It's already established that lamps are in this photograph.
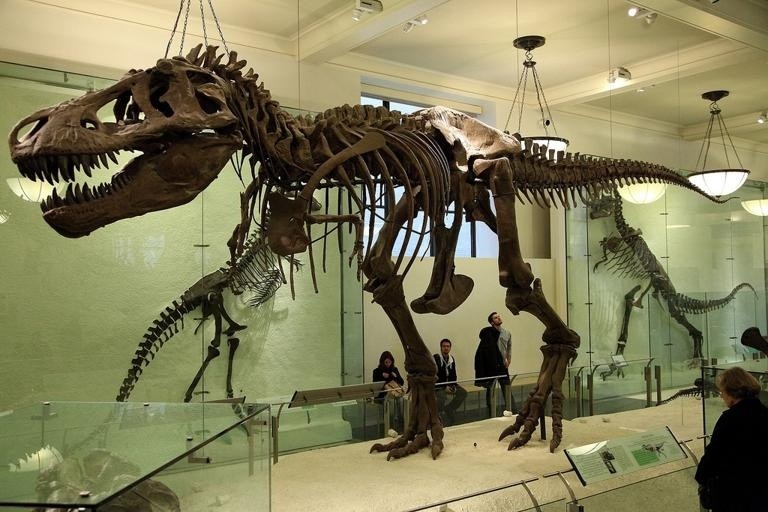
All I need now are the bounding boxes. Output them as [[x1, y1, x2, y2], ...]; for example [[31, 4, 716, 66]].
[[757, 115, 766, 124], [503, 35, 570, 163], [741, 199, 768, 216], [685, 90, 750, 196], [609, 176, 668, 204]]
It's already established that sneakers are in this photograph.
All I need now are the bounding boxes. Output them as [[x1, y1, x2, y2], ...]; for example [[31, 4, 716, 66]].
[[445, 405, 455, 421]]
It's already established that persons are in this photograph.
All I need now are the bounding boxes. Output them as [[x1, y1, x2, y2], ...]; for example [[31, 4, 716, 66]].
[[693, 366, 768, 511], [473, 312, 519, 417], [372, 350, 404, 435], [432, 339, 468, 424]]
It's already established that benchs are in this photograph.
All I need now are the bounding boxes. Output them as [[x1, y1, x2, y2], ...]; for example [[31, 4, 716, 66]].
[[364, 376, 538, 437]]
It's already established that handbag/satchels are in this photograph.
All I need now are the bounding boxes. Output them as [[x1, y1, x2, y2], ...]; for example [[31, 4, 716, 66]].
[[385, 380, 405, 397]]
